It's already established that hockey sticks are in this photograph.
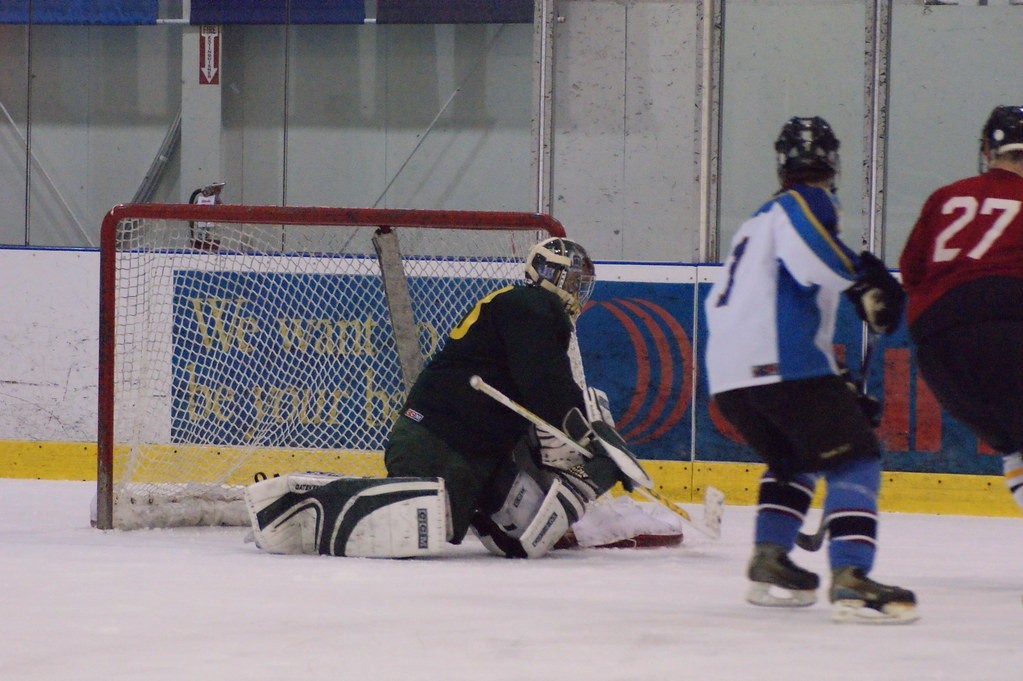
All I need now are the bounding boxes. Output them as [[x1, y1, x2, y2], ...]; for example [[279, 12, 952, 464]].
[[468, 374, 726, 542], [794, 333, 881, 553]]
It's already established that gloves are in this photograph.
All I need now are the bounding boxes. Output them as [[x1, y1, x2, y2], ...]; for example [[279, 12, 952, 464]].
[[583, 444, 637, 491], [843, 265, 902, 334]]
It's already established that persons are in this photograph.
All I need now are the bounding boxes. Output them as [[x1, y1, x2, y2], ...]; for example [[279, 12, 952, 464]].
[[899, 106, 1023, 508], [704, 115, 921, 625], [245, 237, 652, 559]]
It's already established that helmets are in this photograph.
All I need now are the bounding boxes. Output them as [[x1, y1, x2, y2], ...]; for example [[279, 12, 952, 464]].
[[525, 237, 597, 319], [775, 116, 840, 188], [978, 106, 1023, 174]]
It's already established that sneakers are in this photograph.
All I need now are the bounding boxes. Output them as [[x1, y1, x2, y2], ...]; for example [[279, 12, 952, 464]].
[[744, 543, 820, 607], [828, 568, 917, 624]]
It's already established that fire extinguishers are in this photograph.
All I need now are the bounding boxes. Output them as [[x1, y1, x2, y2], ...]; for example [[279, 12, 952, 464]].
[[188, 180, 226, 249]]
